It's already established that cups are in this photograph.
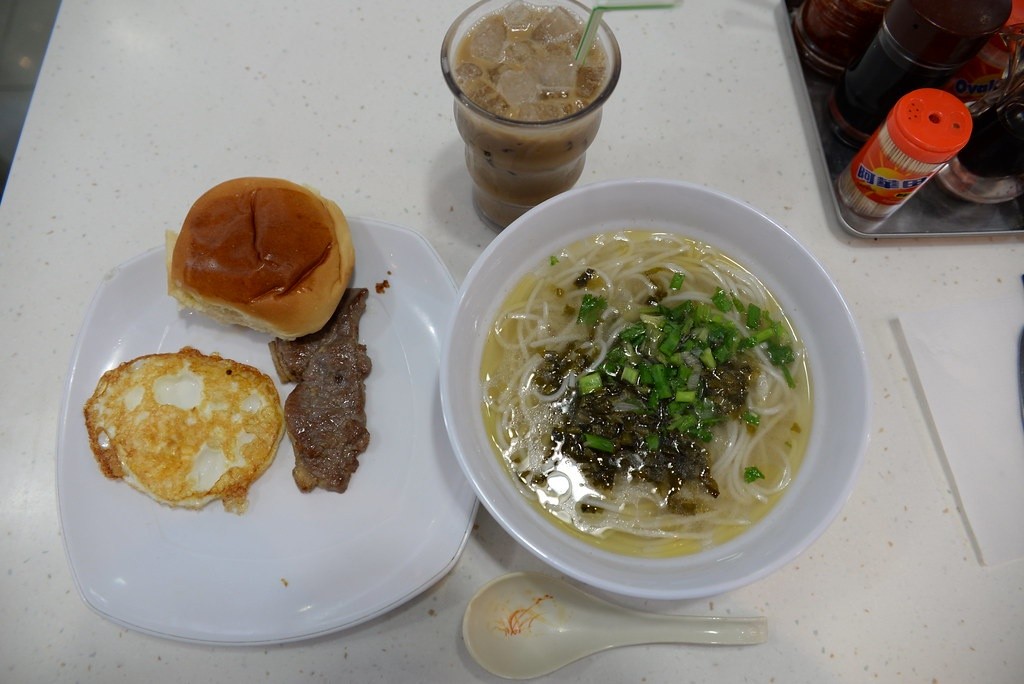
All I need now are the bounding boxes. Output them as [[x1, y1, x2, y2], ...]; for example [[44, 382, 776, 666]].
[[441, 0, 621, 229], [822, 0, 1013, 152], [793, 0, 892, 80]]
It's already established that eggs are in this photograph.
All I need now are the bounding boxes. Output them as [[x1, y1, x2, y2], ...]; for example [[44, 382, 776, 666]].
[[84, 347, 285, 510]]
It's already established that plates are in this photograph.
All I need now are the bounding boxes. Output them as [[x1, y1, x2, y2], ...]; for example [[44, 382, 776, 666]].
[[57, 217, 481, 646]]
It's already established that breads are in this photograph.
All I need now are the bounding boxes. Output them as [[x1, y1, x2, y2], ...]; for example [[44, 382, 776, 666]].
[[167, 175, 353, 337]]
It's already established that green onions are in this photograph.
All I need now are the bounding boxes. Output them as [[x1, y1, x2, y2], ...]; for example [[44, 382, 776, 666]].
[[544, 255, 797, 483]]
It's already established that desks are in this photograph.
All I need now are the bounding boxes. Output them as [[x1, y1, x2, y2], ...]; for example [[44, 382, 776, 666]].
[[0, 0, 1024, 683]]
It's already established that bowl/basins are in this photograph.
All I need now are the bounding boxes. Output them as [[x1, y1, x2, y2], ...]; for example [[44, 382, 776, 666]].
[[439, 179, 868, 600]]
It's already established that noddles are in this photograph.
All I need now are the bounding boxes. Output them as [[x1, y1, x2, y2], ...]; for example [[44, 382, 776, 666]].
[[481, 234, 812, 556]]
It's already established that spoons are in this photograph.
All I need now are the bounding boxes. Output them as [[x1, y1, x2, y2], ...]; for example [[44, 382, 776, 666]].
[[463, 572, 768, 680]]
